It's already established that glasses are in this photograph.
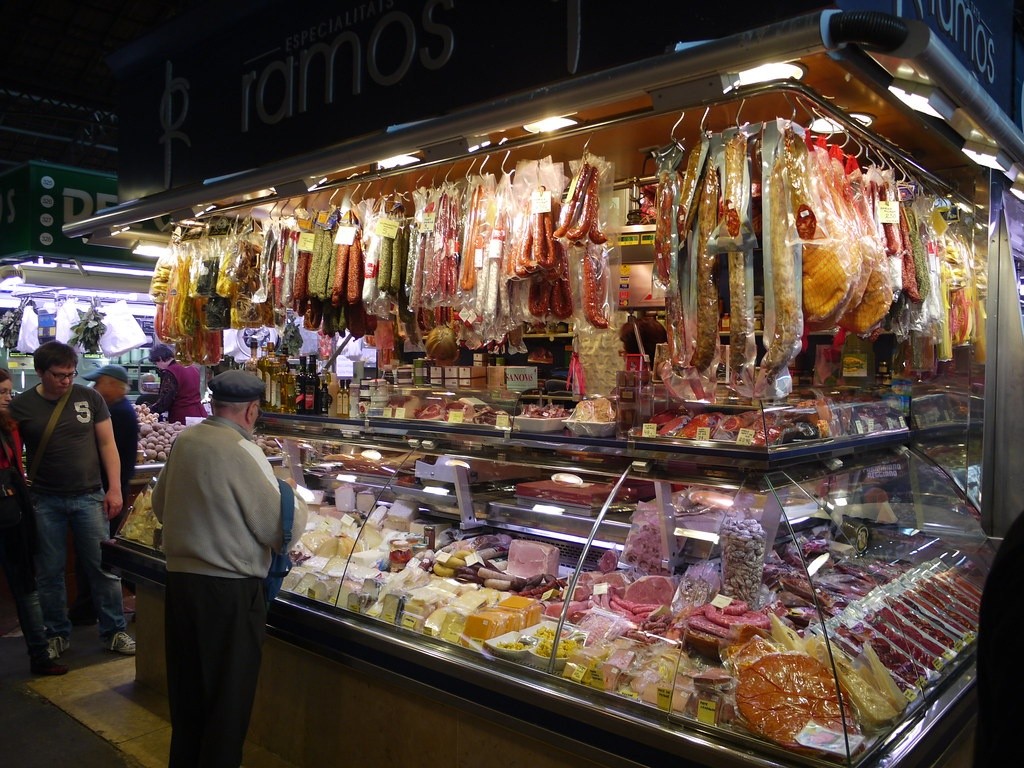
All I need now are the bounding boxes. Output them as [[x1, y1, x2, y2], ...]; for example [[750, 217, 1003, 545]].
[[618, 349, 627, 357], [47, 368, 78, 380]]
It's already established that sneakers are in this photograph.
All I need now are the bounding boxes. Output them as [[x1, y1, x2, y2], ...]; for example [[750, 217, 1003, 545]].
[[100, 631, 136, 656], [46, 636, 71, 659], [30, 658, 69, 676]]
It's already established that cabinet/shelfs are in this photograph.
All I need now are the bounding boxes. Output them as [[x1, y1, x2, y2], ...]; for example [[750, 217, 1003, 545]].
[[1, 387, 1023, 768]]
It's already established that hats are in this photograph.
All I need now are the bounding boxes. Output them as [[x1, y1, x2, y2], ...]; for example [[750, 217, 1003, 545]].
[[81, 364, 128, 385], [208, 369, 269, 405]]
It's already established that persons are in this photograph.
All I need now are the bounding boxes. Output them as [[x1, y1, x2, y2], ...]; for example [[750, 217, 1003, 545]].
[[135, 372, 169, 422], [0, 367, 70, 676], [146, 344, 210, 426], [80, 364, 142, 612], [146, 370, 291, 768], [8, 341, 139, 656]]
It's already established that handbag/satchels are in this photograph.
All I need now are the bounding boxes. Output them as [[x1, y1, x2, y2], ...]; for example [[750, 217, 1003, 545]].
[[0, 432, 22, 533]]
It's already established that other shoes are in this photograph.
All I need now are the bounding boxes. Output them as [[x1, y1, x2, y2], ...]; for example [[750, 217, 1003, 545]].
[[131, 613, 136, 623], [72, 613, 98, 626]]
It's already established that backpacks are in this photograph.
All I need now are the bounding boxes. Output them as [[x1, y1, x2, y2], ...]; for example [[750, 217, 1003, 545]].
[[263, 476, 296, 613]]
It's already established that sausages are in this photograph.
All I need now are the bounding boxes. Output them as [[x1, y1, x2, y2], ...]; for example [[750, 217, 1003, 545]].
[[153, 117, 988, 761]]
